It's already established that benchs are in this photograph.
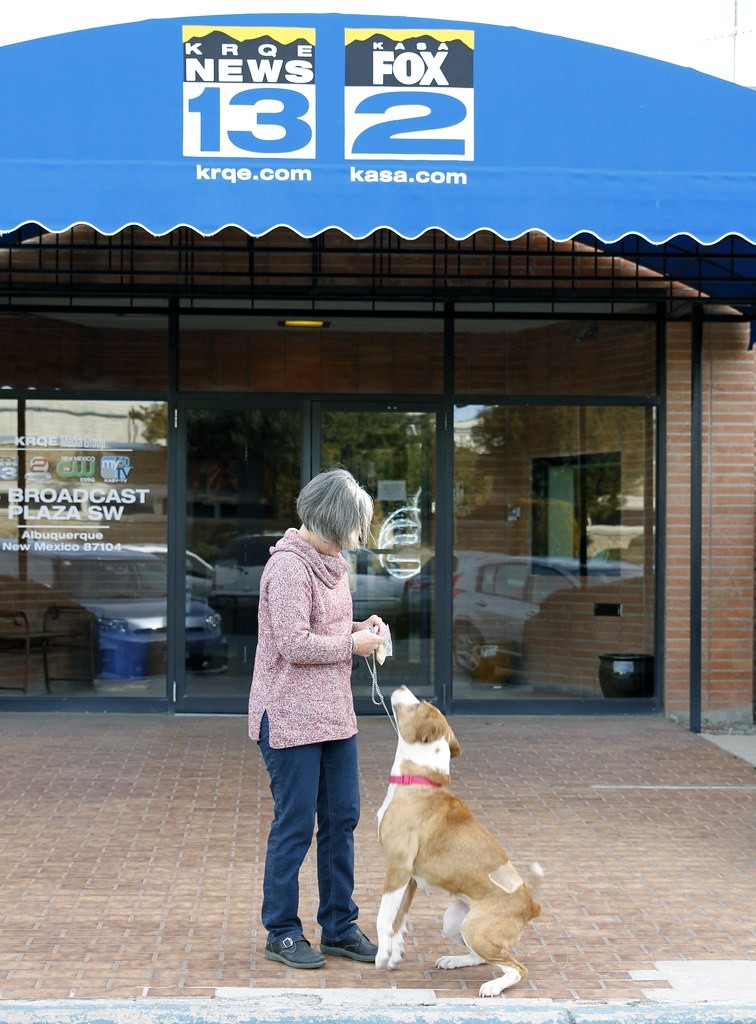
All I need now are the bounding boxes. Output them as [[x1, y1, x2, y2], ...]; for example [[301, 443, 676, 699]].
[[0, 606, 97, 696]]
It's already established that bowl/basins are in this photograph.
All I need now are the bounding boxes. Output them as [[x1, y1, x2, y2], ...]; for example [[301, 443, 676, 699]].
[[598, 653, 656, 698], [465, 640, 514, 686]]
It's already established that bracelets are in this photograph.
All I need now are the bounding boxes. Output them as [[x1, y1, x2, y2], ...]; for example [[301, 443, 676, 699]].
[[350, 634, 358, 654]]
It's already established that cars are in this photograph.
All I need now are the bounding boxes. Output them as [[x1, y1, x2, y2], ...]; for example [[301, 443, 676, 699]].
[[404, 551, 645, 676], [0, 539, 215, 659]]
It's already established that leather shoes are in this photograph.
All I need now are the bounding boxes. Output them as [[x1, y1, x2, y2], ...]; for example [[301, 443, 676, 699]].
[[265, 935, 327, 968], [320, 928, 379, 961]]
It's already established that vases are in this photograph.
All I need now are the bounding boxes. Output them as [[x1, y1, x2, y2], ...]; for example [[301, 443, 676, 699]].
[[468, 640, 513, 688], [598, 652, 654, 698]]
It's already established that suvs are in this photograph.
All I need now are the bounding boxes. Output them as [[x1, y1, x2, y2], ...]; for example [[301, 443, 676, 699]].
[[455, 496, 593, 553], [585, 496, 658, 558], [211, 530, 403, 619]]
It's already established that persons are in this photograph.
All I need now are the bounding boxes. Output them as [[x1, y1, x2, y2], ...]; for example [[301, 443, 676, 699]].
[[248, 468, 394, 969]]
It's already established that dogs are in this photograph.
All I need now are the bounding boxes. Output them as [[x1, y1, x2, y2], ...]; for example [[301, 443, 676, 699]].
[[375, 684, 545, 998]]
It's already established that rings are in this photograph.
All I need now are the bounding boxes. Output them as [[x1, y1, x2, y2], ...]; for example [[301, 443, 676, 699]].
[[368, 627, 375, 633]]
